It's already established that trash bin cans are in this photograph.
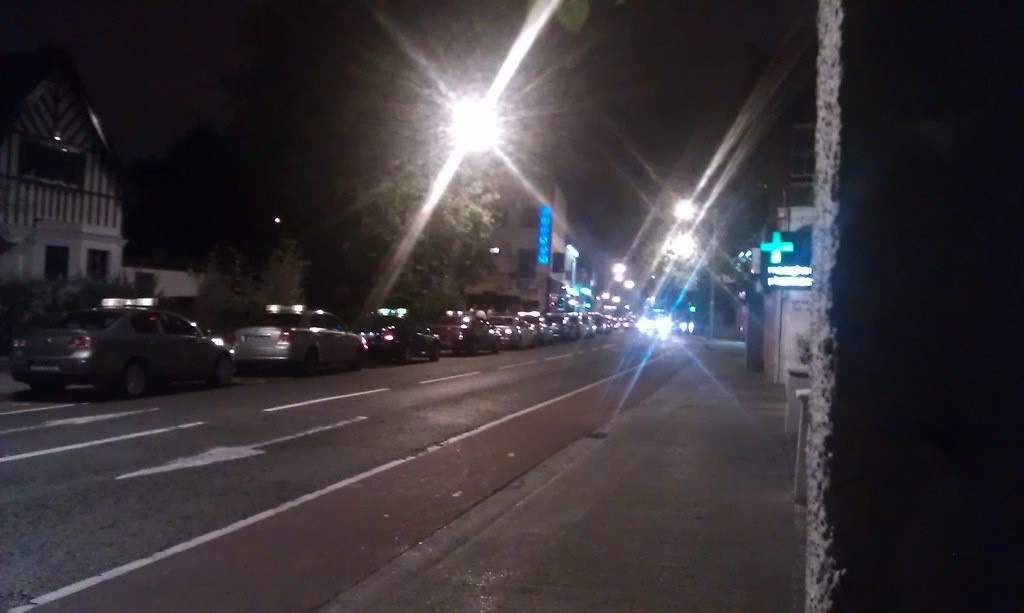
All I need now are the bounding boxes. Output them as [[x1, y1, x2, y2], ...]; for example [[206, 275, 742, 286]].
[[786, 364, 811, 438], [793, 389, 810, 503]]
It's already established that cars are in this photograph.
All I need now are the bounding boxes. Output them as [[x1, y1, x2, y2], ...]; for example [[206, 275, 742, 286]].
[[545, 311, 638, 344], [427, 310, 500, 356], [7, 306, 234, 390], [224, 301, 368, 378], [484, 315, 545, 350], [349, 306, 442, 363], [515, 311, 554, 346]]
